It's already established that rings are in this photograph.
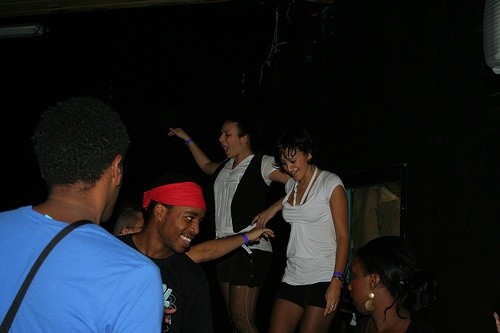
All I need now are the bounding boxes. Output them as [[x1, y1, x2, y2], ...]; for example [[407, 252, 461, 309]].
[[330, 305, 335, 308]]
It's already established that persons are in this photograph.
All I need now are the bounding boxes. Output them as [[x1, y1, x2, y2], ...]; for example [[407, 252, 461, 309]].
[[271, 126, 349, 333], [0, 97, 163, 333], [113, 175, 500, 333], [169, 115, 295, 333]]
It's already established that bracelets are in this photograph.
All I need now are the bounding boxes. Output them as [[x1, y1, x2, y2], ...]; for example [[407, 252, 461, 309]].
[[239, 233, 249, 244], [186, 138, 192, 145], [332, 271, 345, 283]]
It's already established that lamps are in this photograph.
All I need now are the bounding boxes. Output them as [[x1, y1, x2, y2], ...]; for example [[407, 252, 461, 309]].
[[483, 0, 500, 95]]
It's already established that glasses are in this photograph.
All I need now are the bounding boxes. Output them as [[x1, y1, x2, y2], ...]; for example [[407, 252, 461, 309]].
[[119, 225, 145, 234]]
